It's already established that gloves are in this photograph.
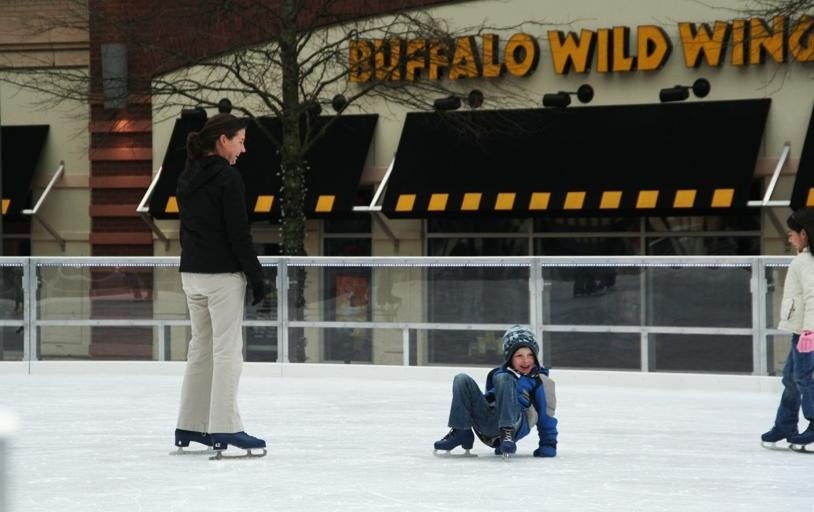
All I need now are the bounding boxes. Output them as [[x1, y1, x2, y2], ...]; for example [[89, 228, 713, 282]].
[[252, 280, 269, 306], [533, 441, 558, 458], [795, 330, 813, 355]]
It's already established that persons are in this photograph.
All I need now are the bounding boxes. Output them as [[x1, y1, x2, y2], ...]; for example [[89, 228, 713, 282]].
[[432, 321, 559, 460], [170, 111, 273, 450], [752, 205, 814, 447]]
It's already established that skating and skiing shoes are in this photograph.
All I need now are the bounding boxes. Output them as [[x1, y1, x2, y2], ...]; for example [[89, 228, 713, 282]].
[[497, 426, 517, 454], [432, 428, 474, 450]]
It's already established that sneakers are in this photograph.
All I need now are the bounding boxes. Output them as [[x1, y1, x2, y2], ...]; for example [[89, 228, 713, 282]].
[[789, 423, 814, 444], [173, 428, 213, 446], [762, 428, 799, 442], [211, 431, 267, 450]]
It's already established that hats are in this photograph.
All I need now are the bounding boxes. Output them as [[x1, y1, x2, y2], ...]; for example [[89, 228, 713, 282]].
[[498, 322, 541, 367]]
